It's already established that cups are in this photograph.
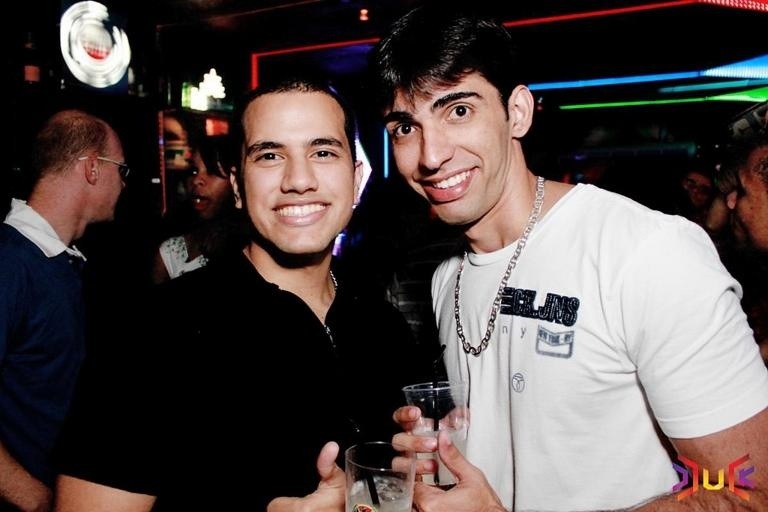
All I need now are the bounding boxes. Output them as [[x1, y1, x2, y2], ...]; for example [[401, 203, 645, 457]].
[[402, 382, 469, 442], [344, 442, 416, 512]]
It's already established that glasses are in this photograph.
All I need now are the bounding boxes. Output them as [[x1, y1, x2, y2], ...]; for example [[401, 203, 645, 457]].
[[78, 156, 129, 178]]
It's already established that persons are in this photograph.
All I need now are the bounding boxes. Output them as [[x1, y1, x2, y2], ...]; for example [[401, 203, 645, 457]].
[[149, 132, 243, 316], [377, 9, 766, 510], [0, 114, 768, 512], [56, 79, 457, 512]]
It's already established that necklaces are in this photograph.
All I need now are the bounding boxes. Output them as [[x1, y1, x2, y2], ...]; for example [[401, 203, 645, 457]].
[[322, 265, 344, 347], [442, 175, 560, 357]]
